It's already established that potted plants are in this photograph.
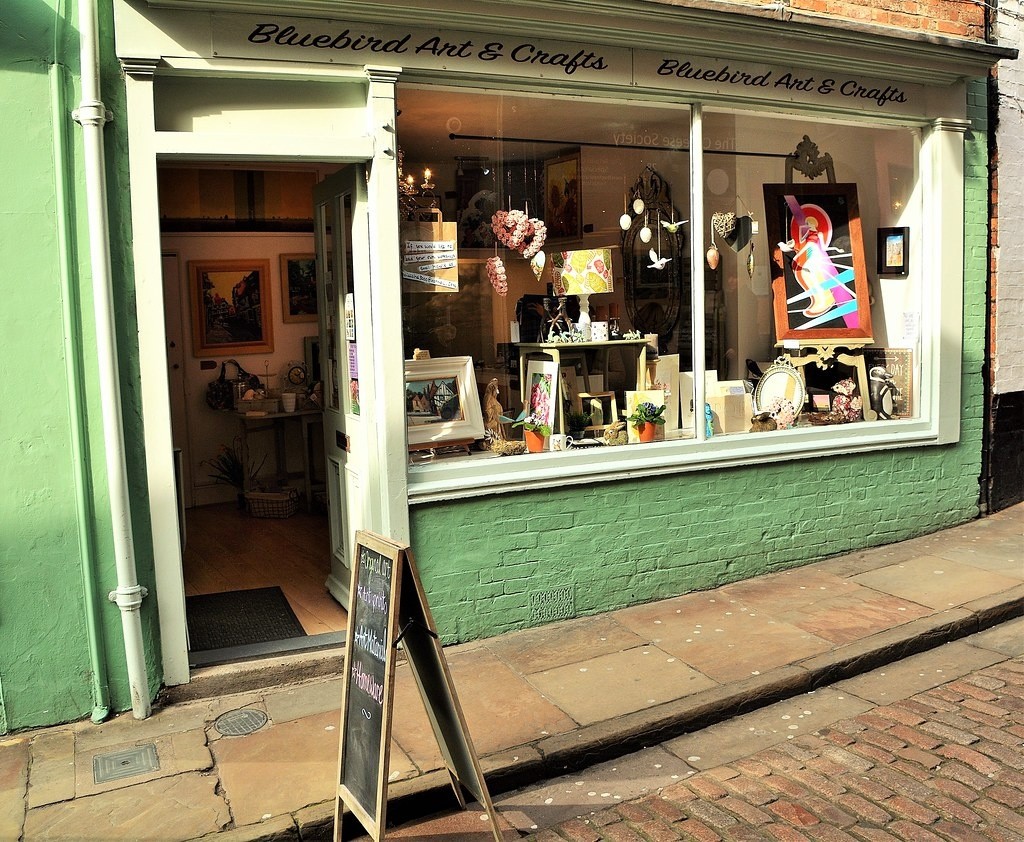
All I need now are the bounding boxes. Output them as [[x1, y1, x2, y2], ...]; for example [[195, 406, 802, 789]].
[[200, 442, 272, 511]]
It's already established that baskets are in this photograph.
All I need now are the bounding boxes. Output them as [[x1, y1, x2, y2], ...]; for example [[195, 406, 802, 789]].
[[237, 398, 280, 413], [243, 486, 299, 518]]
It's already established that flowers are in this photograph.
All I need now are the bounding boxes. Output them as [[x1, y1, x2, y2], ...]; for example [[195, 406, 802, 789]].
[[511, 413, 551, 438], [625, 402, 667, 427]]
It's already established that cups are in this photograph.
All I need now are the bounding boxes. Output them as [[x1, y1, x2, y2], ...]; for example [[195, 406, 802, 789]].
[[549, 434, 574, 452], [585, 321, 609, 342], [282, 393, 295, 412], [644, 333, 659, 359], [570, 323, 589, 343]]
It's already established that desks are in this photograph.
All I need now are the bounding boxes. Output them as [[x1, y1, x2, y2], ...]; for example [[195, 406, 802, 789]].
[[235, 409, 330, 515], [514, 338, 651, 448]]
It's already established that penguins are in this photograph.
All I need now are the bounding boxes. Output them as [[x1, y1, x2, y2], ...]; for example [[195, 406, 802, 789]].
[[869, 366, 903, 420]]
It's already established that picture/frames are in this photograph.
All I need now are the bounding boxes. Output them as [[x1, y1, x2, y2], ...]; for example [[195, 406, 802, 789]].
[[404, 354, 488, 443], [876, 226, 908, 275], [190, 258, 276, 359], [761, 183, 873, 339], [542, 153, 583, 245], [523, 360, 558, 445], [280, 251, 334, 323]]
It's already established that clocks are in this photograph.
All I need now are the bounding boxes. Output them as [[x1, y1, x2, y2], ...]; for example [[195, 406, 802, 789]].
[[283, 361, 310, 393]]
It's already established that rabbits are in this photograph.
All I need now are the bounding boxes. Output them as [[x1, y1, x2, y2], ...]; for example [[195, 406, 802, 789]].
[[603, 419, 629, 446], [749, 412, 778, 433]]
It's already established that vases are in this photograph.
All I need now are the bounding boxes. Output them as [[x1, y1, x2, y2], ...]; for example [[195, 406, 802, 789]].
[[524, 430, 543, 453], [637, 422, 656, 443]]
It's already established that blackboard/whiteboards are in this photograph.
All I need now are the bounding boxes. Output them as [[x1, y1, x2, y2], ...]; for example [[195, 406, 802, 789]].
[[335, 530, 496, 842]]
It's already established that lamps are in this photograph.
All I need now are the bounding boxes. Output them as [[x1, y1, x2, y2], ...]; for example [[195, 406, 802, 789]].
[[397, 109, 440, 223], [563, 248, 613, 340], [456, 159, 463, 175], [481, 162, 490, 175]]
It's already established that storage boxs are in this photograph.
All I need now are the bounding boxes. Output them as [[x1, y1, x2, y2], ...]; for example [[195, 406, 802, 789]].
[[236, 397, 281, 414], [246, 489, 299, 519]]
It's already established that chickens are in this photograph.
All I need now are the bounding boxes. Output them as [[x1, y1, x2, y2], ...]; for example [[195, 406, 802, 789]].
[[481, 378, 506, 439]]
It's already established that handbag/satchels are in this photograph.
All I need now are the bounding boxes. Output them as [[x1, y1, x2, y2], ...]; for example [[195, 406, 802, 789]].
[[206, 360, 260, 410]]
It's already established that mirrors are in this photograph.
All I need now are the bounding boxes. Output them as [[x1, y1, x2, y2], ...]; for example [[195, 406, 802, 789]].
[[620, 167, 682, 341]]
